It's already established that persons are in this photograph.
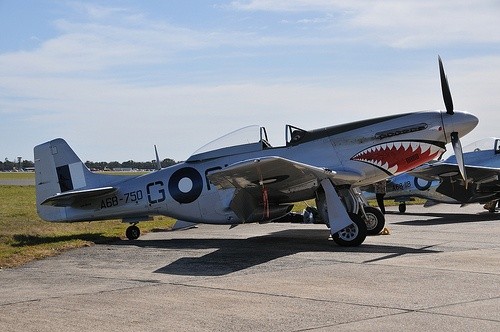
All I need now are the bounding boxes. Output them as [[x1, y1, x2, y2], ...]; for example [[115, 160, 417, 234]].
[[373, 179, 386, 214]]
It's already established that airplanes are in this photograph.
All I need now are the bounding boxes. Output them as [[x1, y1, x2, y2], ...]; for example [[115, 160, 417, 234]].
[[34, 55, 476, 247], [355, 138, 500, 221]]
